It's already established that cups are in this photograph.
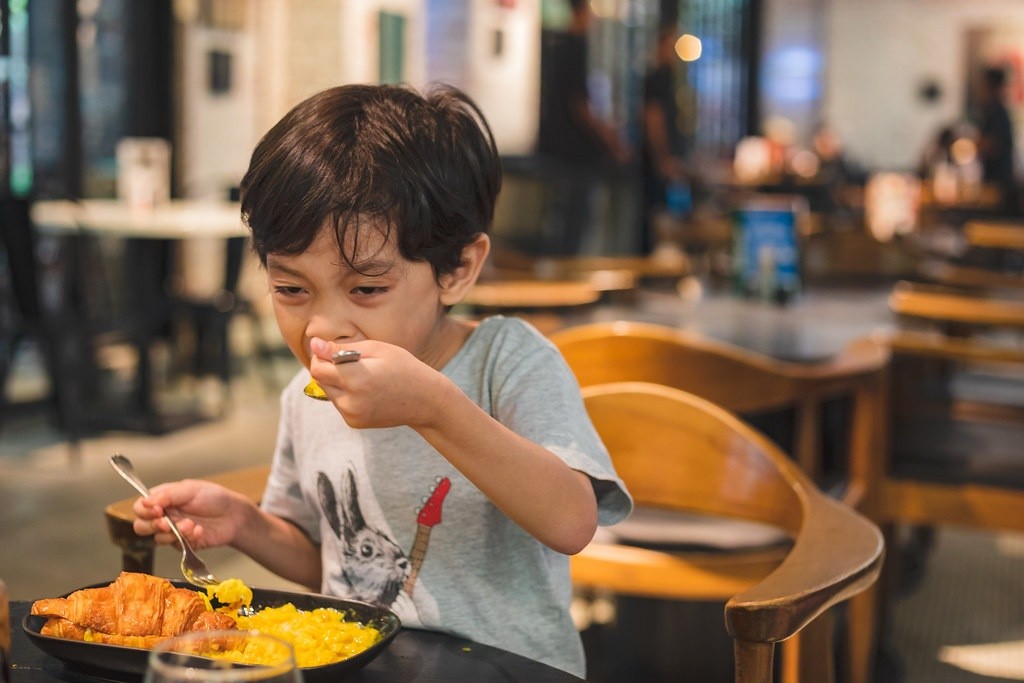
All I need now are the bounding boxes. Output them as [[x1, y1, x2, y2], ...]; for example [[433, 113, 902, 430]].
[[142, 629, 305, 683]]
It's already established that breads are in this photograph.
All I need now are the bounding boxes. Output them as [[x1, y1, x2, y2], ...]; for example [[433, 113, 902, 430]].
[[27, 571, 247, 652]]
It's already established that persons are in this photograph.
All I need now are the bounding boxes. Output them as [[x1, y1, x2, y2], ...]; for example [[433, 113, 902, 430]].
[[540, 0, 1024, 277], [131, 84, 636, 675]]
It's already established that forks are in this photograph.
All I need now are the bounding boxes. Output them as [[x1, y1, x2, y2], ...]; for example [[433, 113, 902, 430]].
[[107, 451, 222, 591]]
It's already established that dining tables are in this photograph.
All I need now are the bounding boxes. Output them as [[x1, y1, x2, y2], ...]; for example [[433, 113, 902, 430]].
[[30, 196, 263, 435], [0, 599, 589, 683]]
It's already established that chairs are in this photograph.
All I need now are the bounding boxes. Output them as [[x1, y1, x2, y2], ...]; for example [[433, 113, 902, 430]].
[[463, 176, 1021, 526], [579, 382, 889, 683]]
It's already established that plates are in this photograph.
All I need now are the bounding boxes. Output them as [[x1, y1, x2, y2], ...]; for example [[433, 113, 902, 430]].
[[22, 574, 403, 683]]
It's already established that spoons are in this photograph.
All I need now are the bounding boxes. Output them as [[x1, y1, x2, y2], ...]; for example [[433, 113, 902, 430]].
[[302, 348, 364, 402]]
